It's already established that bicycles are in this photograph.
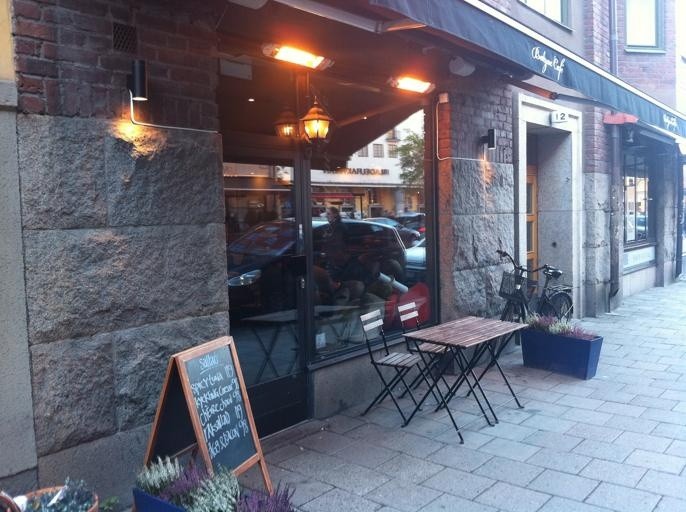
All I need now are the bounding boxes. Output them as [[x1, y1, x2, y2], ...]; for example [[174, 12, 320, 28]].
[[489, 250, 573, 368]]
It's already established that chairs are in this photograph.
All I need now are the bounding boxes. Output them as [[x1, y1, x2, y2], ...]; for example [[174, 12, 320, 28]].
[[398, 302, 455, 404], [360, 309, 422, 424]]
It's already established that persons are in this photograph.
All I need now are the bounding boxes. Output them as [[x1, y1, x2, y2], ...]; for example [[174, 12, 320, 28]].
[[309, 250, 352, 316], [318, 206, 355, 289]]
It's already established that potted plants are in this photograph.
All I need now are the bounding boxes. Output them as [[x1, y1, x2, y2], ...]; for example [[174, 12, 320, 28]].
[[520, 311, 603, 380], [133, 455, 297, 512], [8, 475, 100, 512]]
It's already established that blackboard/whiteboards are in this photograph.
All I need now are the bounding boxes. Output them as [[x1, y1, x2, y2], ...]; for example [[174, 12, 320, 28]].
[[176, 335, 261, 478]]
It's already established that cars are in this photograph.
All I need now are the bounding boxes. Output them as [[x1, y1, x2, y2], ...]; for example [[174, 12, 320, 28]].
[[226, 212, 427, 327]]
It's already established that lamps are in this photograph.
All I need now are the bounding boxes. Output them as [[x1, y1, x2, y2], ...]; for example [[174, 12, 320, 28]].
[[300, 96, 333, 142], [126, 57, 148, 104], [483, 128, 495, 150]]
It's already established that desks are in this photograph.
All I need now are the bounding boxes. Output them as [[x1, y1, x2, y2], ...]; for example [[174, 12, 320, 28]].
[[401, 315, 529, 444]]
